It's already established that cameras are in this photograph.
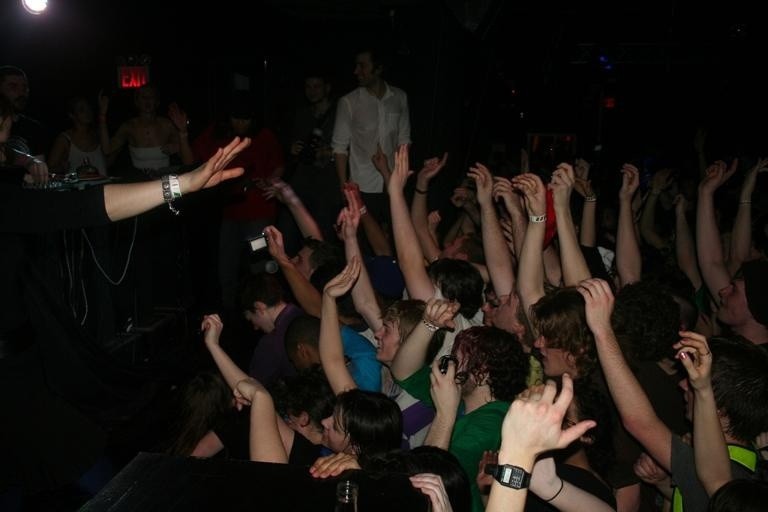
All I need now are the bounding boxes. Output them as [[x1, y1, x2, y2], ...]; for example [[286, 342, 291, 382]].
[[247, 236, 267, 252], [439, 354, 459, 375]]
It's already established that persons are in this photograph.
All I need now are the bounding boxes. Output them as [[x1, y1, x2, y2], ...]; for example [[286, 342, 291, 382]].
[[3, 43, 766, 511]]
